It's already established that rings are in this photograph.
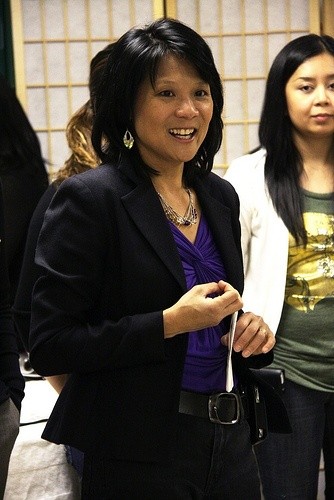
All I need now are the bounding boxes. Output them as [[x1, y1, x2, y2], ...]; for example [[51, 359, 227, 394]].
[[260, 329, 267, 336]]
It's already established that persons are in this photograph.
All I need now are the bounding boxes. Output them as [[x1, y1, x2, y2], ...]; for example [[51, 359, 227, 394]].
[[26, 19, 276, 500], [0, 77, 50, 500], [12, 96, 111, 390], [223, 31, 334, 500]]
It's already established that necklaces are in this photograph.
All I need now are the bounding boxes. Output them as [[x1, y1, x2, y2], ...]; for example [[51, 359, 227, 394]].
[[159, 187, 197, 228]]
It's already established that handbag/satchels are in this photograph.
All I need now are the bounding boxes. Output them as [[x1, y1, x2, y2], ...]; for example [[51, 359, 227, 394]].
[[244, 369, 294, 445]]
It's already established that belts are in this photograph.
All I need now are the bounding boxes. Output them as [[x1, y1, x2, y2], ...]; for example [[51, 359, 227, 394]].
[[176, 389, 246, 427]]
